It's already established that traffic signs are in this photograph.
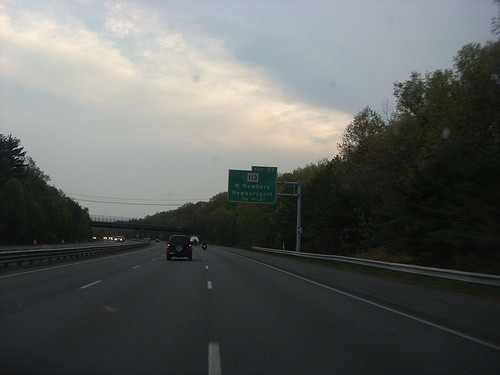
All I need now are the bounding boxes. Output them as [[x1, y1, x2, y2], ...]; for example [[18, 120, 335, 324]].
[[228, 166, 278, 205]]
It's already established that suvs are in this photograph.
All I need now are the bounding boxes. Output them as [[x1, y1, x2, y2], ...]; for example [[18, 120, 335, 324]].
[[166, 235, 193, 261], [114, 236, 126, 243], [190, 236, 200, 246]]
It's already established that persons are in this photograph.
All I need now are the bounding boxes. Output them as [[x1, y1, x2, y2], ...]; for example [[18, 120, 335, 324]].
[[201, 238, 208, 247]]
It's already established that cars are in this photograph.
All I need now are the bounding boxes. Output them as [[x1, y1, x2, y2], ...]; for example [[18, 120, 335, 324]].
[[155, 239, 160, 243]]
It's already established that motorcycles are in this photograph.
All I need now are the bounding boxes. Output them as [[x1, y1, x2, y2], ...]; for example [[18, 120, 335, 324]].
[[201, 242, 208, 251]]
